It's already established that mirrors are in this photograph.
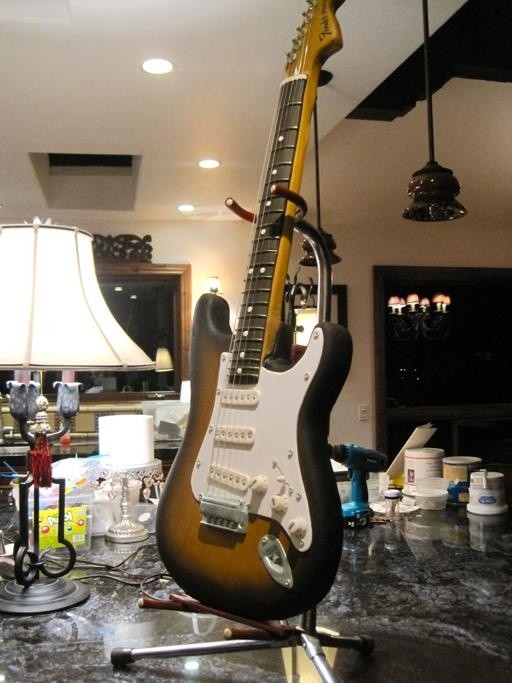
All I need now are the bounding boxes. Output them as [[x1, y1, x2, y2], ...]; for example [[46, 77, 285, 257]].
[[2, 259, 194, 406], [283, 282, 349, 366]]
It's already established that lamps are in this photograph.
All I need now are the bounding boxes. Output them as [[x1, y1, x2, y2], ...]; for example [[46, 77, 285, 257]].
[[154, 345, 175, 372], [382, 291, 454, 344], [400, 0, 468, 223], [293, 305, 320, 346]]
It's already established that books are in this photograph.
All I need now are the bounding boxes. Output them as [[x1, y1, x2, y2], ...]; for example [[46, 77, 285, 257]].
[[385, 420, 439, 477]]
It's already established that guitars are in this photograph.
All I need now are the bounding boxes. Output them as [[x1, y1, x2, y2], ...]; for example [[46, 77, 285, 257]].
[[154, 0, 352, 623]]
